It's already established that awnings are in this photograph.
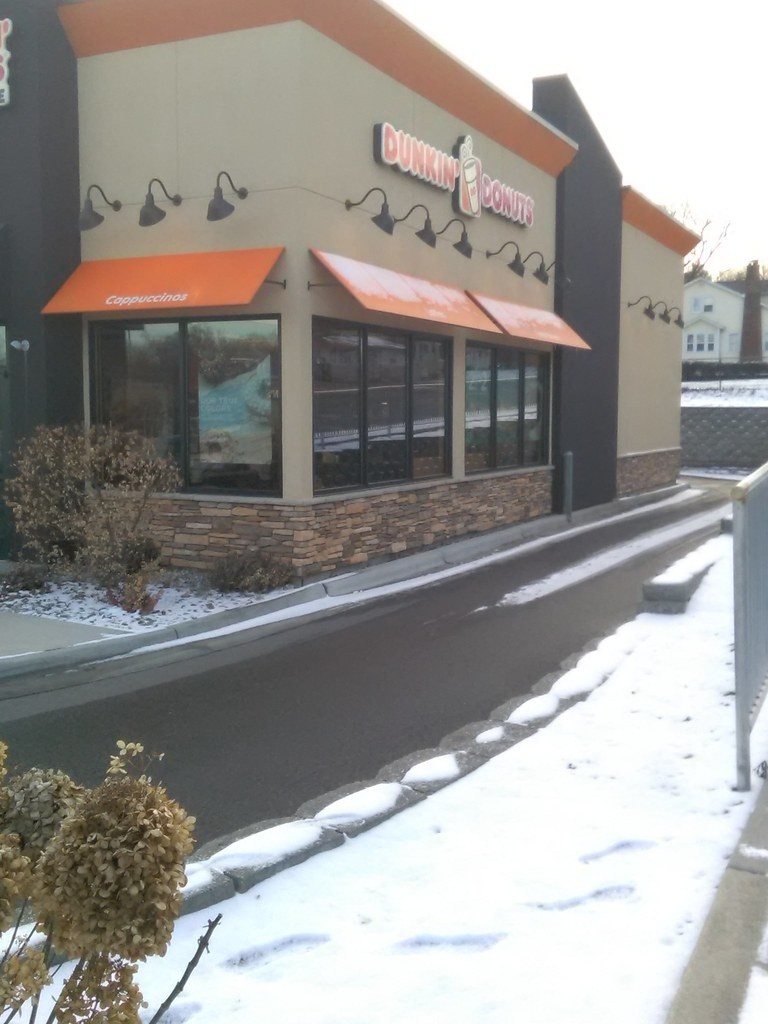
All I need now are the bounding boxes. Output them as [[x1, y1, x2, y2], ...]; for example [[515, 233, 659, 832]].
[[38, 242, 287, 318], [306, 247, 504, 334], [464, 287, 593, 352]]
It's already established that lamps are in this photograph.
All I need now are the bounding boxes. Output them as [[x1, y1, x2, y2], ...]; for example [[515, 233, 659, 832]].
[[643, 300, 671, 325], [138, 178, 183, 227], [394, 203, 437, 250], [486, 241, 525, 279], [659, 307, 684, 330], [435, 218, 473, 260], [205, 171, 249, 221], [628, 295, 656, 321], [77, 184, 122, 232], [345, 186, 395, 236], [522, 250, 549, 287]]
